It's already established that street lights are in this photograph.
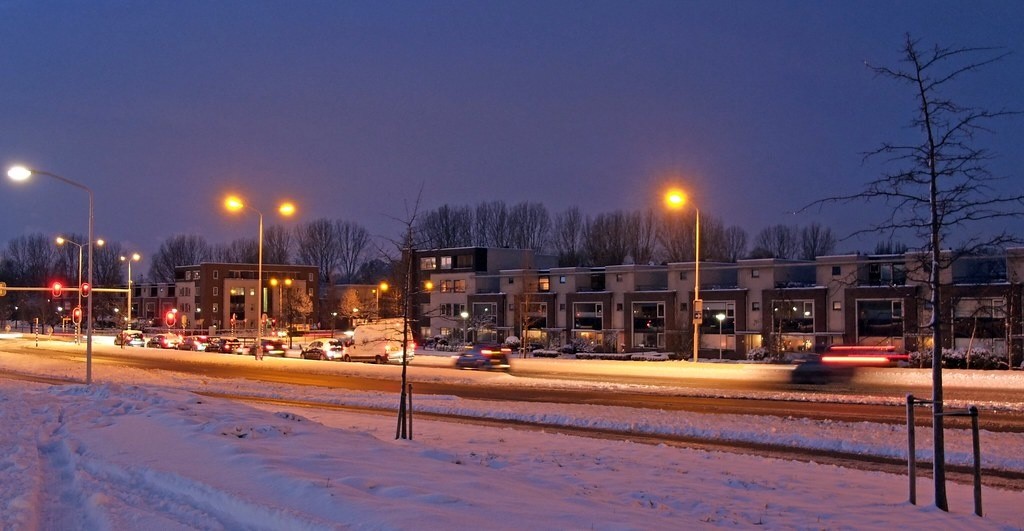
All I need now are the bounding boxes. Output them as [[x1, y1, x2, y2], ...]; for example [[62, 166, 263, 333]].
[[7, 163, 94, 384], [55, 236, 104, 344], [119, 252, 140, 330], [222, 194, 296, 361], [666, 190, 702, 363]]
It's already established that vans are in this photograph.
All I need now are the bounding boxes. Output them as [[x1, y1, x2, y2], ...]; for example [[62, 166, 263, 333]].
[[343, 317, 416, 364]]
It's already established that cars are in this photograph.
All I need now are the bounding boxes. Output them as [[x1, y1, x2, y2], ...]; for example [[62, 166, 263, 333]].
[[455, 341, 510, 372], [114, 330, 145, 347], [178, 336, 212, 351], [299, 338, 344, 361], [147, 333, 179, 349], [249, 337, 287, 357], [205, 337, 243, 354]]
